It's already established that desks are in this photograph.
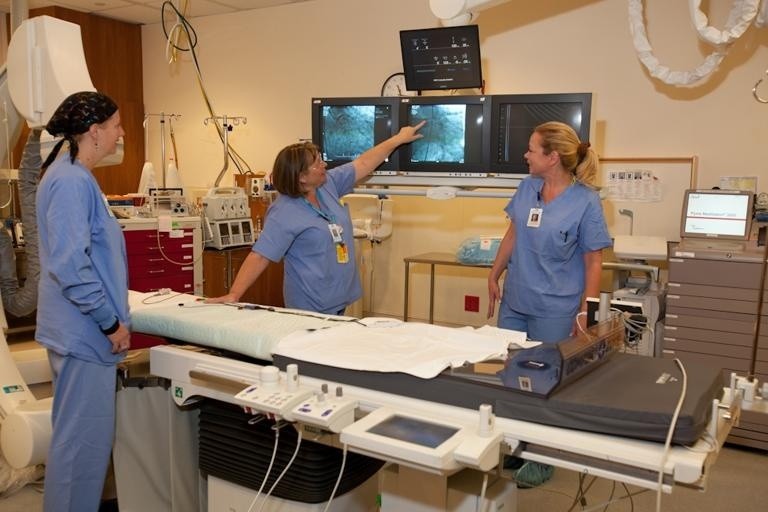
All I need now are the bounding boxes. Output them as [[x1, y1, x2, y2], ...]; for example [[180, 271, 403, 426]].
[[403, 251, 506, 324]]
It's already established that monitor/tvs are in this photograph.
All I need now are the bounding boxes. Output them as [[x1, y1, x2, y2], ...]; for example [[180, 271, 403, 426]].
[[311, 96, 398, 170], [681, 189, 754, 253], [399, 26, 483, 91], [399, 96, 492, 171], [492, 92, 592, 172]]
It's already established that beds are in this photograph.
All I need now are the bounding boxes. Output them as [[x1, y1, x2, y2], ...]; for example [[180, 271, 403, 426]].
[[118, 287, 758, 511]]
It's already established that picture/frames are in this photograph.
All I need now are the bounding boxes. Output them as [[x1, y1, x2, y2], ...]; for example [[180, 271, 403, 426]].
[[598, 155, 698, 270]]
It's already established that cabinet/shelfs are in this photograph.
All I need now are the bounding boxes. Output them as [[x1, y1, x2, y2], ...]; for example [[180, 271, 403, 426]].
[[202, 191, 285, 308], [661, 243, 768, 450], [117, 216, 205, 350]]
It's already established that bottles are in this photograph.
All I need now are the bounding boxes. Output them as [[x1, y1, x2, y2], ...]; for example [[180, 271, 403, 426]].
[[166, 158, 180, 189], [137, 160, 155, 194]]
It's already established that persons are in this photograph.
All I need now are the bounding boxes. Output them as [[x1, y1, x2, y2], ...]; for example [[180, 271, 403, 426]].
[[33, 90, 132, 512], [203, 120, 431, 314], [532, 215, 538, 222], [332, 229, 338, 238], [485, 121, 614, 490]]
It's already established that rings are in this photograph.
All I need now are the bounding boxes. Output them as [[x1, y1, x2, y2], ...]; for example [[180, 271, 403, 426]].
[[121, 345, 128, 349]]
